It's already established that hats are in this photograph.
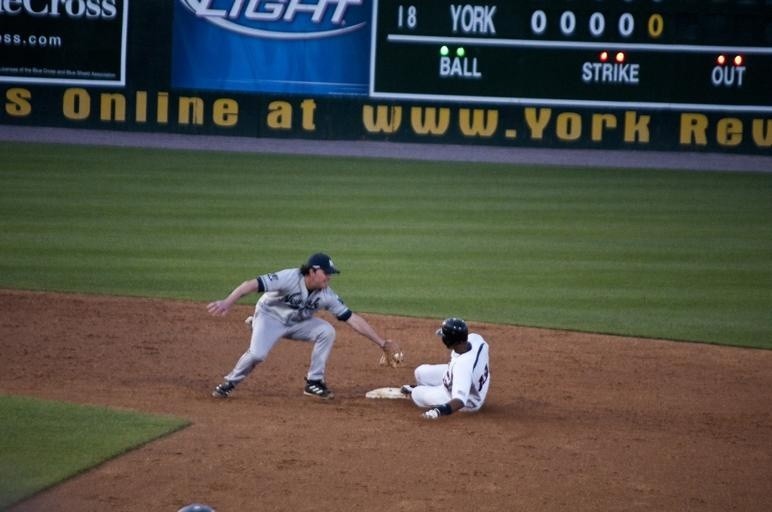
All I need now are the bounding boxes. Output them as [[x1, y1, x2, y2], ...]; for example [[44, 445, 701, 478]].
[[305, 252, 340, 275]]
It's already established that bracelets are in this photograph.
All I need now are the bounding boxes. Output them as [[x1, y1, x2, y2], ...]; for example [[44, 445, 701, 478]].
[[435, 404, 452, 415]]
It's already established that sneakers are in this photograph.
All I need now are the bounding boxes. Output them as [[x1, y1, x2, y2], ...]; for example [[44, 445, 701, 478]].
[[401, 382, 417, 394], [210, 382, 235, 398], [302, 380, 335, 400]]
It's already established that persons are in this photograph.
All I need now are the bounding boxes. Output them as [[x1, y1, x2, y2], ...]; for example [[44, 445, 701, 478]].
[[207, 253, 394, 401], [400, 318, 491, 420]]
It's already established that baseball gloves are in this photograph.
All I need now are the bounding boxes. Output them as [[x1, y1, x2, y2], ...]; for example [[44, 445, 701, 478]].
[[380, 338, 408, 368]]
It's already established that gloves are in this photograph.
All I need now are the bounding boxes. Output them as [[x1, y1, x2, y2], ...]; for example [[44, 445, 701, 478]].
[[422, 407, 440, 420]]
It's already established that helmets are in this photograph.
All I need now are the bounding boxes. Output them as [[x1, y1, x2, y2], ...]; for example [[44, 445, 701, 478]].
[[436, 317, 469, 340]]
